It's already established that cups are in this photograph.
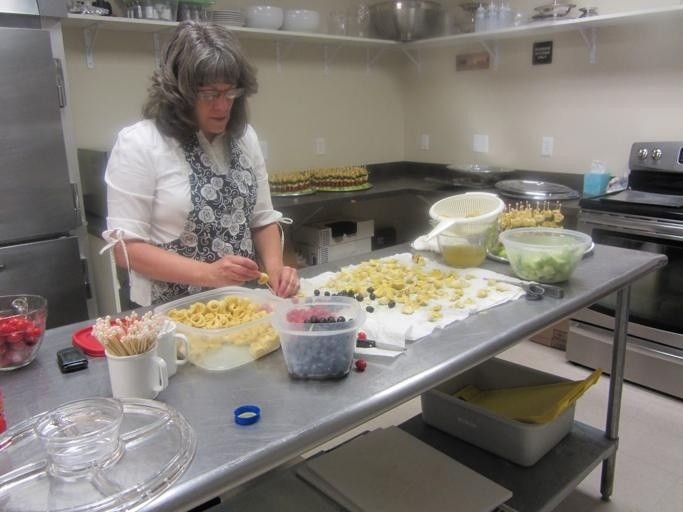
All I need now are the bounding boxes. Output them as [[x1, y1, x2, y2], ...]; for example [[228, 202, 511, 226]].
[[102, 318, 190, 402], [36, 397, 123, 470]]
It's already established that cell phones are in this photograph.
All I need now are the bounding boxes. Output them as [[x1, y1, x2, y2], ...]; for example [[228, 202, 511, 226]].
[[56, 347, 88, 374]]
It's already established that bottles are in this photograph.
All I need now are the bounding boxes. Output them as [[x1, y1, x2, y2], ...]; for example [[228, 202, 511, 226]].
[[474, 2, 513, 31], [125, 1, 207, 21]]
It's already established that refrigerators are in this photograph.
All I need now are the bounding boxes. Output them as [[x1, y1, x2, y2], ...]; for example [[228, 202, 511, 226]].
[[1, 27, 89, 339]]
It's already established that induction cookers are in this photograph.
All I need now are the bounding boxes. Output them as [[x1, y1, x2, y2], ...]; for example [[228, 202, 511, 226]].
[[582, 188, 683, 210]]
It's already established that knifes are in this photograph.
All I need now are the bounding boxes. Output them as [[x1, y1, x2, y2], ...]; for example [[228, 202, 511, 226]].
[[357, 340, 408, 352]]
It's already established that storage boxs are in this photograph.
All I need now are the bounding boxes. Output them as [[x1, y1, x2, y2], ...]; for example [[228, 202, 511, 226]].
[[420, 357, 576, 468]]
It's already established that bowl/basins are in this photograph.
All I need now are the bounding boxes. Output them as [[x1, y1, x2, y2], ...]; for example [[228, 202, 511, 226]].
[[538, 5, 572, 16], [244, 5, 320, 32], [428, 222, 490, 269], [0, 293, 50, 375], [579, 7, 600, 17], [498, 226, 595, 284], [367, 0, 442, 41]]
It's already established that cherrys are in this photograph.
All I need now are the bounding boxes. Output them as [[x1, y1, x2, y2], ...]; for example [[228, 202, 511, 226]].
[[0, 316, 42, 366]]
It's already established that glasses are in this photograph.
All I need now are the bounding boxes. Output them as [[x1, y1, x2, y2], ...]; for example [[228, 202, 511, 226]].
[[195, 85, 245, 102]]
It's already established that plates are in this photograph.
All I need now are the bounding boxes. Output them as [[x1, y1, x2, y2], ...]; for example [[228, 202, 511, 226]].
[[207, 9, 246, 26]]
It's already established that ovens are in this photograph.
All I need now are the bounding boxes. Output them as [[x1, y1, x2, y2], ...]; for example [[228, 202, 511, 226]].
[[566, 209, 682, 403]]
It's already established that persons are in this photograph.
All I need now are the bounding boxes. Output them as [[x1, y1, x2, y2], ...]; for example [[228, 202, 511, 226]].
[[100, 19, 303, 312]]
